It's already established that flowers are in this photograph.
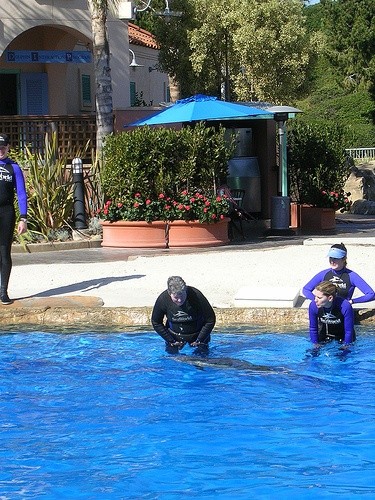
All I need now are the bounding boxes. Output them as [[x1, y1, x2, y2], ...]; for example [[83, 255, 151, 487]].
[[95, 186, 234, 223], [314, 186, 353, 212]]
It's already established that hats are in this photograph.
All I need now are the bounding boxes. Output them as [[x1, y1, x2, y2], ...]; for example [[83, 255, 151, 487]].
[[0, 135, 10, 146], [326, 247, 346, 259]]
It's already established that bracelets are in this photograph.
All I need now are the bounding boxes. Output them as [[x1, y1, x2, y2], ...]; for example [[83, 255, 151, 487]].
[[20, 219, 28, 224]]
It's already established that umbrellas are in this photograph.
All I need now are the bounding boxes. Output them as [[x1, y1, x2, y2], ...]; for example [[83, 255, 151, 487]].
[[123, 94, 275, 198]]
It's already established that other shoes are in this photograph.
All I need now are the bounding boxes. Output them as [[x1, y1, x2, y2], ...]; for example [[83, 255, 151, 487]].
[[0, 288, 13, 305]]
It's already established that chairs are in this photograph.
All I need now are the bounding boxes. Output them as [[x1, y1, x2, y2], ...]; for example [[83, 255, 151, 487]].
[[230, 189, 246, 240]]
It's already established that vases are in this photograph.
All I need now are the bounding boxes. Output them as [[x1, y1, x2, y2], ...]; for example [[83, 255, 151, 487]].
[[99, 220, 167, 248], [167, 216, 231, 248], [301, 206, 337, 231]]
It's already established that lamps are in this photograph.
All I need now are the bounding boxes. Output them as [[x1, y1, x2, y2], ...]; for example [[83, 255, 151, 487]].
[[129, 48, 144, 68], [133, 0, 183, 17]]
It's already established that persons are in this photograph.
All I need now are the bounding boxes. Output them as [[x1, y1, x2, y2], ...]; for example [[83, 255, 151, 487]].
[[302, 243, 375, 305], [0, 134, 28, 305], [151, 276, 216, 352], [308, 280, 356, 343]]
